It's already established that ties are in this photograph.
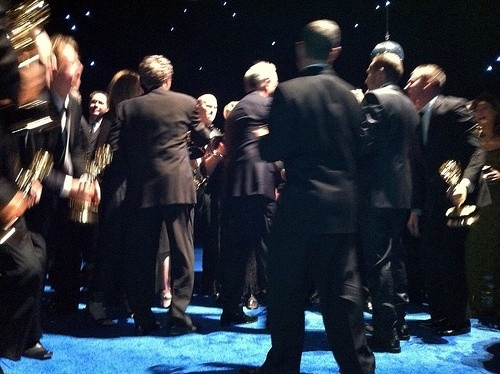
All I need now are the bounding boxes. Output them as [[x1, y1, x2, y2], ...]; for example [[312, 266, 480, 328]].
[[418, 112, 424, 146]]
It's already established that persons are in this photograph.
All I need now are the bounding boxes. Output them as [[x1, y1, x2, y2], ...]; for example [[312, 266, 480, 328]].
[[251, 19, 375, 374], [0, 36, 500, 361]]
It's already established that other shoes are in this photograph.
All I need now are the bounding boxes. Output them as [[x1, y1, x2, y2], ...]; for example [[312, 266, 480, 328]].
[[167, 318, 202, 336], [23, 341, 53, 360], [140, 316, 160, 330], [221, 309, 258, 327], [239, 365, 262, 374]]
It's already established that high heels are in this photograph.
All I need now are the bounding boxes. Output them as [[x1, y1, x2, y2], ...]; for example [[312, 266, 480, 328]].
[[87, 300, 115, 326]]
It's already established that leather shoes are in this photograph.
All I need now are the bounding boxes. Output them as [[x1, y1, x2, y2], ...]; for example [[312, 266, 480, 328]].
[[432, 319, 470, 336], [417, 315, 447, 328], [364, 330, 401, 353], [397, 318, 410, 340]]
